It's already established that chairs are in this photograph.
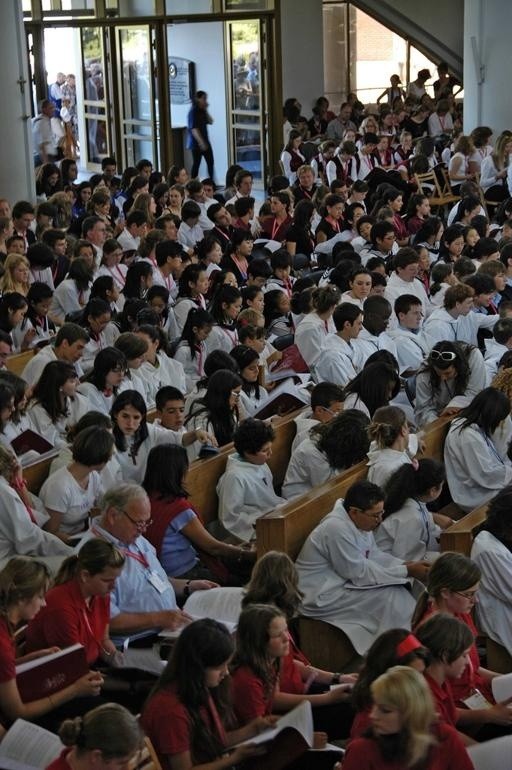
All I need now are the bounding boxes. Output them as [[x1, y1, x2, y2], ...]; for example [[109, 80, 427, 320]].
[[440, 168, 458, 205], [473, 172, 503, 215], [414, 170, 454, 220]]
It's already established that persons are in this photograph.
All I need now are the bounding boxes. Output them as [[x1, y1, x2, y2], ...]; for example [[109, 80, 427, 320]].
[[30, 99, 61, 169], [47, 72, 67, 147], [376, 74, 406, 105], [278, 93, 511, 219], [406, 68, 432, 104], [0, 158, 510, 769], [61, 74, 77, 159], [232, 51, 261, 161], [432, 61, 463, 101], [184, 89, 215, 192], [85, 61, 105, 162]]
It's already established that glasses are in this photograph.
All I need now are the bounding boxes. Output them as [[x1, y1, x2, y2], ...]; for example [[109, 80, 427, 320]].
[[429, 349, 457, 362], [231, 390, 241, 398], [113, 505, 154, 528]]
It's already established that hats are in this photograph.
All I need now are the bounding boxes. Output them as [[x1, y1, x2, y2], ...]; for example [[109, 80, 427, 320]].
[[236, 66, 249, 76]]
